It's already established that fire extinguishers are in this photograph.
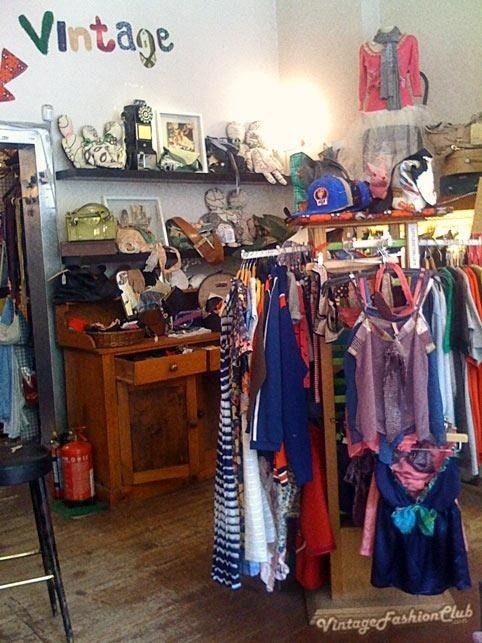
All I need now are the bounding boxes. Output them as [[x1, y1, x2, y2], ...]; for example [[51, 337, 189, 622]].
[[46, 430, 71, 501], [60, 426, 96, 509]]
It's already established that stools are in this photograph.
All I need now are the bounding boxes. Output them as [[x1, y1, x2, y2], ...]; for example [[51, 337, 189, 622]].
[[0, 439, 74, 643]]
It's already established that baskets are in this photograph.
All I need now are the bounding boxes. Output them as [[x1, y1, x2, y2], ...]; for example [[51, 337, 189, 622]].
[[83, 328, 147, 349]]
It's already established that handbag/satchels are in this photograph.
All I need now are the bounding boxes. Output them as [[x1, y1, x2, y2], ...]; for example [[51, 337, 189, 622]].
[[65, 202, 117, 243], [390, 147, 438, 213]]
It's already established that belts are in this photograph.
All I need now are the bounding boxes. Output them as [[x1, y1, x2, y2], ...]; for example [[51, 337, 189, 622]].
[[165, 215, 225, 266]]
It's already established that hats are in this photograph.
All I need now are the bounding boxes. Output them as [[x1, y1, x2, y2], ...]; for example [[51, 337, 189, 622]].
[[291, 173, 372, 217]]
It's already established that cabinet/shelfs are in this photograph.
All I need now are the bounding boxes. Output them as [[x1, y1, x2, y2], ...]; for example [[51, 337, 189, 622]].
[[57, 299, 222, 510], [225, 208, 482, 629], [57, 164, 296, 260]]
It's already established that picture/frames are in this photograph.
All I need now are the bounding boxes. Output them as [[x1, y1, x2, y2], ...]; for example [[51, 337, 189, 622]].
[[156, 111, 209, 173], [103, 195, 169, 249]]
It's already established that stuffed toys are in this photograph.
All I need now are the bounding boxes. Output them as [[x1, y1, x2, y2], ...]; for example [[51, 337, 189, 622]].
[[227, 119, 288, 186], [366, 161, 389, 200], [197, 187, 256, 248]]
